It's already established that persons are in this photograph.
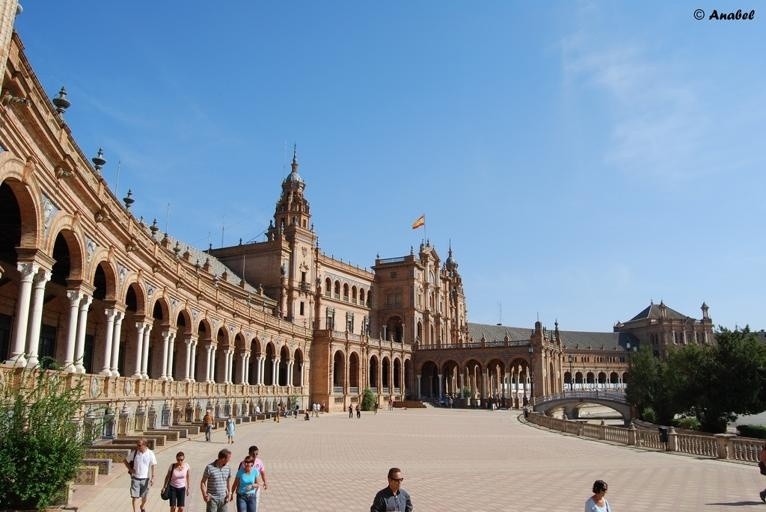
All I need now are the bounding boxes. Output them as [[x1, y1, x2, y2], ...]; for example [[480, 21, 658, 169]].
[[200, 448, 233, 511], [374, 400, 378, 413], [758, 445, 766, 503], [161, 451, 190, 511], [229, 455, 260, 512], [433, 392, 528, 410], [237, 445, 269, 511], [389, 399, 393, 412], [369, 468, 413, 511], [254, 401, 361, 424], [226, 413, 236, 444], [202, 409, 215, 441], [584, 479, 611, 511], [123, 437, 156, 511]]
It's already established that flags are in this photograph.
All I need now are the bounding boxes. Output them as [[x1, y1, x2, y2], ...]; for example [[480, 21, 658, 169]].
[[411, 215, 424, 230]]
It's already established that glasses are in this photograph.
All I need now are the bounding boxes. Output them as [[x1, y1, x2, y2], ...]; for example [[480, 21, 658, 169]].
[[390, 478, 403, 481]]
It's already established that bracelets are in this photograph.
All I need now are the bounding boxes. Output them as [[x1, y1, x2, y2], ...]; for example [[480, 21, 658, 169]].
[[204, 495, 208, 499], [251, 484, 254, 489]]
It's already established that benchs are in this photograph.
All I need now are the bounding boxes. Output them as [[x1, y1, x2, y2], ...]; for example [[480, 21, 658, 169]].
[[48, 407, 307, 507]]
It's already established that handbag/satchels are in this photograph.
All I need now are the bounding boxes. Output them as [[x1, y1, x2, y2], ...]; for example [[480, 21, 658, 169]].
[[161, 485, 169, 499], [128, 461, 134, 474]]
